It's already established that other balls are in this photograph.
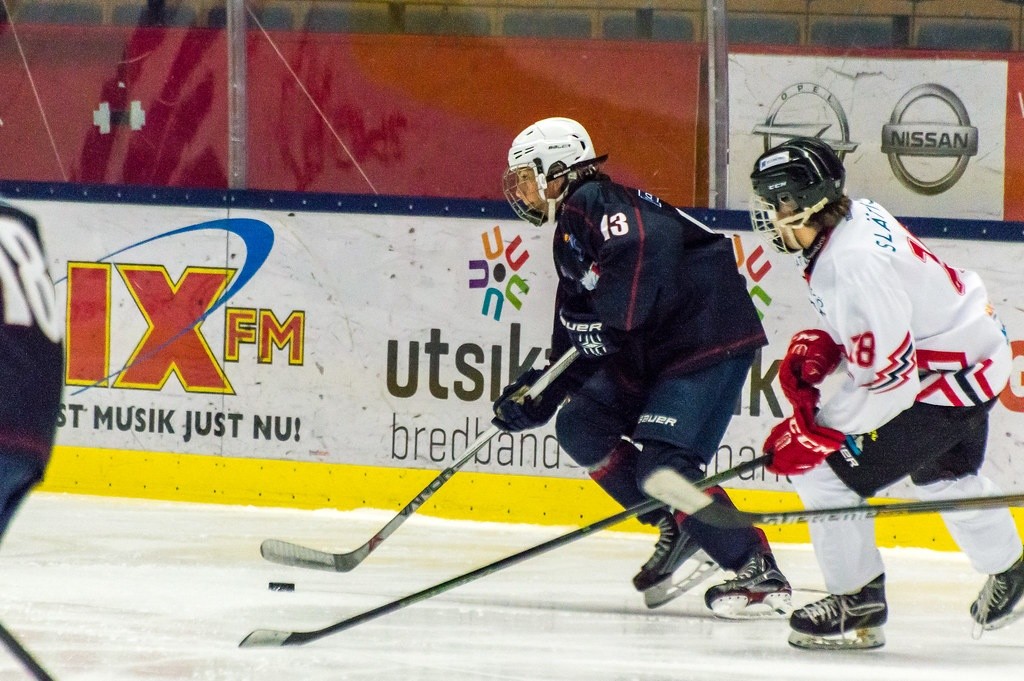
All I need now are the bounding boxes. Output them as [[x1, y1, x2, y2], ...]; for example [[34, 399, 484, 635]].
[[268, 582, 295, 592]]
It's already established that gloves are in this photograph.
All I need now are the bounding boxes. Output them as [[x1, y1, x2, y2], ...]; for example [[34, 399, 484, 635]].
[[492, 365, 566, 431], [780, 329, 843, 407], [560, 296, 624, 358], [763, 407, 845, 475]]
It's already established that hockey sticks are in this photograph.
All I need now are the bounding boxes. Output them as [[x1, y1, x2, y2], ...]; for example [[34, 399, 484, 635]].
[[0, 623, 54, 681], [238, 452, 772, 648], [259, 344, 579, 573], [639, 464, 1023, 530]]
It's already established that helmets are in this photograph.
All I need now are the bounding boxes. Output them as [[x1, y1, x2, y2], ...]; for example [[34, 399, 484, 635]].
[[750, 137, 847, 207], [508, 116, 596, 202]]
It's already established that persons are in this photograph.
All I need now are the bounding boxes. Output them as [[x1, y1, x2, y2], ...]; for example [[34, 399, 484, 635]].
[[749, 138, 1023, 650], [492, 116, 794, 620], [0, 196, 67, 544]]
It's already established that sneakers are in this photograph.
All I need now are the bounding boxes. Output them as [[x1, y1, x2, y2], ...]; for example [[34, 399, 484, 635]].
[[788, 573, 888, 649], [705, 553, 793, 619], [969, 547, 1024, 641], [633, 513, 722, 609]]
